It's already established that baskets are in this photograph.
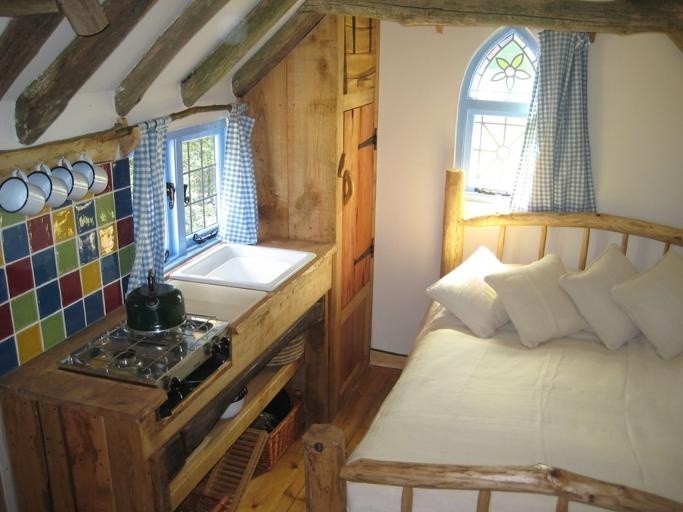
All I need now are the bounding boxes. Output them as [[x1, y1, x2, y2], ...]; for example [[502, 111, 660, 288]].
[[260, 394, 306, 468]]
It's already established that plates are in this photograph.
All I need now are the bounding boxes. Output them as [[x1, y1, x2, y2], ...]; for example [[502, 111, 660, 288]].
[[266, 332, 305, 367]]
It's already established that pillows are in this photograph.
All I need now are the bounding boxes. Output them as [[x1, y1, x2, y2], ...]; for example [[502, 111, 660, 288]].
[[610, 249, 683, 361], [485, 253, 587, 347], [424, 247, 511, 337], [559, 242, 643, 351]]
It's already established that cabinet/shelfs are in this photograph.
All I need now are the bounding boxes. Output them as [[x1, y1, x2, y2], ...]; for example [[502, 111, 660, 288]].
[[238, 13, 380, 424]]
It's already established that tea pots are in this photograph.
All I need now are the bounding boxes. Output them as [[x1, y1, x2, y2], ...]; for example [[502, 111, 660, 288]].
[[126, 268, 188, 334]]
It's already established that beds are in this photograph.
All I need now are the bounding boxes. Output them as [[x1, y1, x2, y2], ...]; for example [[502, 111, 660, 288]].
[[301, 168, 683, 512]]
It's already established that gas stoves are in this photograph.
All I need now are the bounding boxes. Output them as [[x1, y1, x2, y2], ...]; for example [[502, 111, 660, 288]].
[[57, 311, 231, 421]]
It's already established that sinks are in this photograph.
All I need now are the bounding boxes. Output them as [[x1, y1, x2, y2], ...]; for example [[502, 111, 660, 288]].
[[169, 242, 317, 293]]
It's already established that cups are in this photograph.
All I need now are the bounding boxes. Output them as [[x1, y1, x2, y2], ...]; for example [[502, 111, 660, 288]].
[[51, 155, 89, 202], [71, 150, 109, 194], [26, 161, 68, 207], [0, 168, 47, 217]]
